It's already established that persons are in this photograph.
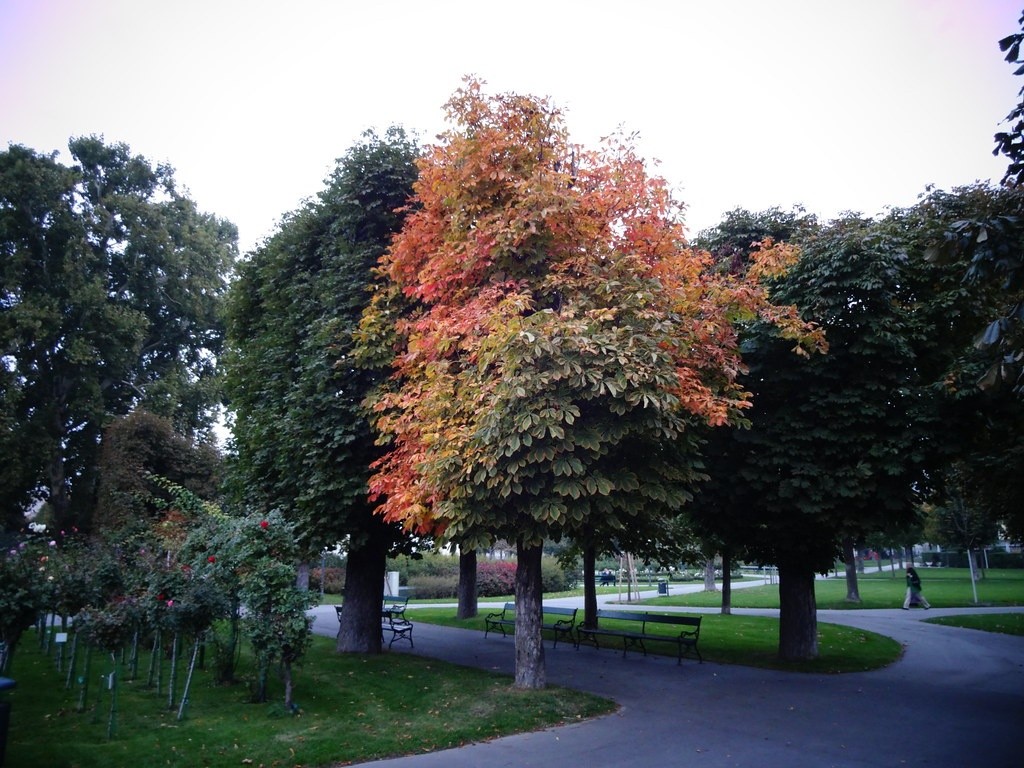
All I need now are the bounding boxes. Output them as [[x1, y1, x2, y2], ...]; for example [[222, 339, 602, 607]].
[[600, 569, 616, 585], [903, 562, 931, 610]]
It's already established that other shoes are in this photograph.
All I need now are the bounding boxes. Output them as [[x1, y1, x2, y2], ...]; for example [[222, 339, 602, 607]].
[[925, 607, 931, 610], [902, 608, 909, 610]]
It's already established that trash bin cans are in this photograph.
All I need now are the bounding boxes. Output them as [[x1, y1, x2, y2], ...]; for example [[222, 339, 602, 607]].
[[658, 582, 667, 593]]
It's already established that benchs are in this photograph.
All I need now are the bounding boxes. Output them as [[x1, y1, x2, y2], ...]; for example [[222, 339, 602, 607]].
[[484, 602, 578, 649], [382, 596, 409, 624], [575, 608, 703, 666], [333, 605, 414, 649], [595, 575, 615, 586]]
